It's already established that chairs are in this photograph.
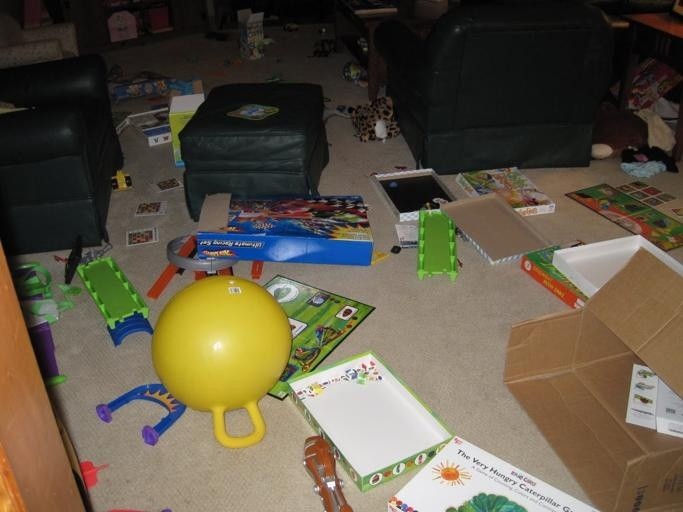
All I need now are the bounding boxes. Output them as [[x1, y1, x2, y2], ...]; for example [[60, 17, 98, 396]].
[[0, 13, 126, 256], [374, 0, 621, 176]]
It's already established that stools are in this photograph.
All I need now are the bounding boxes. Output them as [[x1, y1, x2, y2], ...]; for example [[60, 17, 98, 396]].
[[178, 81, 329, 223]]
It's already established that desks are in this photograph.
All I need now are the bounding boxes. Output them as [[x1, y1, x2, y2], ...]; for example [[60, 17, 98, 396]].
[[621, 11, 683, 165]]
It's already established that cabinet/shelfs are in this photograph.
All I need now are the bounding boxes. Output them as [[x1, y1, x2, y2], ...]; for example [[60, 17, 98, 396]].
[[62, 0, 207, 55]]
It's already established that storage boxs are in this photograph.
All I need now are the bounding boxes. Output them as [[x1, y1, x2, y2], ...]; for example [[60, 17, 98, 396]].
[[238, 8, 265, 61], [195, 192, 375, 266], [167, 80, 207, 167], [502, 247, 683, 512]]
